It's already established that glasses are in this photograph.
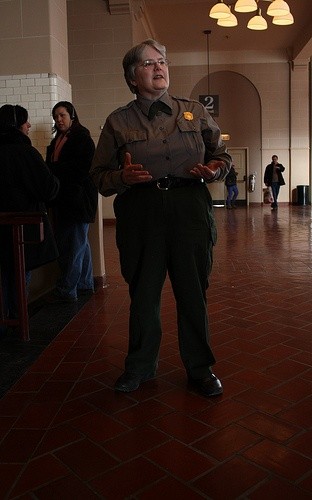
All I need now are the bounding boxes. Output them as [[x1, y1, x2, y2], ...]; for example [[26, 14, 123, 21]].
[[136, 58, 168, 70]]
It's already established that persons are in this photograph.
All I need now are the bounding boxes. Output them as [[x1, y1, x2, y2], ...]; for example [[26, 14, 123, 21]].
[[89, 38, 234, 398], [225, 164, 239, 209], [40, 101, 99, 305], [0, 104, 60, 336], [264, 155, 285, 208]]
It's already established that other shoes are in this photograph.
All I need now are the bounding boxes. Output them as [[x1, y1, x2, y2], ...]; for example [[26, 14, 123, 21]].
[[41, 292, 77, 304], [76, 289, 96, 296]]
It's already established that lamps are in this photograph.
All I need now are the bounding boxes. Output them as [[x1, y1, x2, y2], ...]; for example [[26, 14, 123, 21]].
[[208, 0, 295, 33]]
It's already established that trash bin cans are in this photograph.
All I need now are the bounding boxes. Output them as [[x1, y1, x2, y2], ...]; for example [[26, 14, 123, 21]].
[[297, 185, 310, 206]]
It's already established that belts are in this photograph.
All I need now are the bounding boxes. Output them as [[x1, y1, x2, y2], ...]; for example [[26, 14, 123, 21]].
[[127, 176, 205, 189]]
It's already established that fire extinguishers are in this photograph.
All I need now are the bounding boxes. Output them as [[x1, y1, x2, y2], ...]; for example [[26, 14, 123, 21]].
[[249, 175, 256, 192]]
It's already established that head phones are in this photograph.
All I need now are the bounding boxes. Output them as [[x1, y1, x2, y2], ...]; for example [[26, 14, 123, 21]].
[[11, 107, 17, 129], [71, 109, 75, 120]]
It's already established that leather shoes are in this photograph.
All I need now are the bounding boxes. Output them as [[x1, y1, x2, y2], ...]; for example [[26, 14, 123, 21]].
[[114, 366, 156, 392], [186, 370, 224, 396]]
